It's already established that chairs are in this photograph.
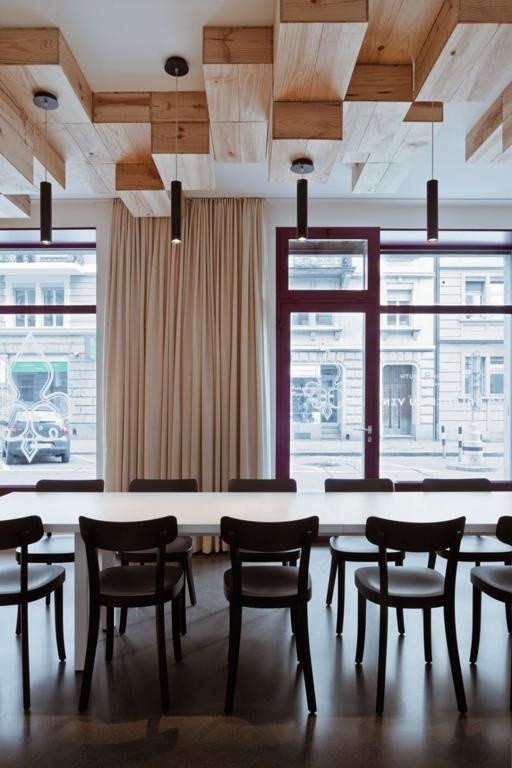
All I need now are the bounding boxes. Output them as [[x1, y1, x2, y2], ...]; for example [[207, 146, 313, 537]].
[[225, 478, 303, 634], [323, 478, 408, 637], [218, 515, 323, 716], [353, 515, 469, 720], [0, 515, 67, 713], [12, 478, 106, 636], [76, 513, 189, 716], [115, 478, 199, 636], [467, 514, 511, 667], [422, 478, 511, 569]]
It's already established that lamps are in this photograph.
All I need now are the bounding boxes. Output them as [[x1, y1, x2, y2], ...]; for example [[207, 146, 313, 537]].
[[290, 157, 315, 243], [163, 54, 190, 246], [424, 102, 440, 245], [32, 88, 61, 246]]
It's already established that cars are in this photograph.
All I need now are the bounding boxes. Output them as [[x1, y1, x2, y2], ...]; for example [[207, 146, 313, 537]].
[[0, 403, 70, 464]]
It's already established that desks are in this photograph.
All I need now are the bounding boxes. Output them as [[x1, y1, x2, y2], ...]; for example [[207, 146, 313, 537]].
[[1, 490, 512, 673]]
